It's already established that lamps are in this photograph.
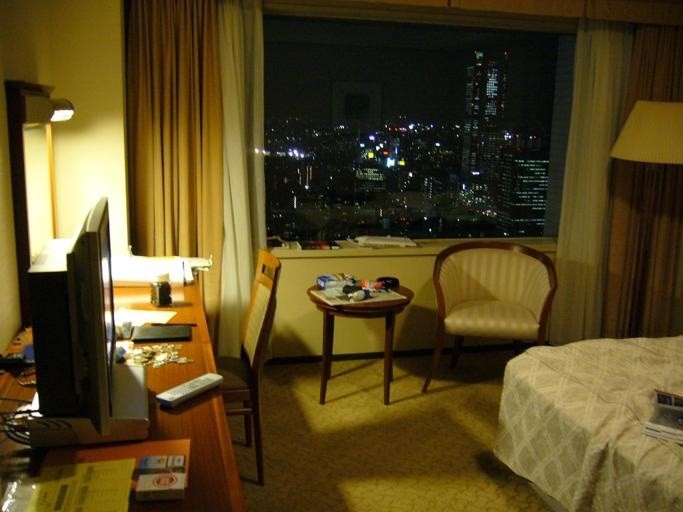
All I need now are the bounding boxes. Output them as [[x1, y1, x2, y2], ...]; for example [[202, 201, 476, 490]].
[[25, 94, 74, 124], [610, 100, 683, 336]]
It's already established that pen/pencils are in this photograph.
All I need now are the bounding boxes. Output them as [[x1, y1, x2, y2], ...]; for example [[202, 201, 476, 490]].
[[150, 323, 197, 326]]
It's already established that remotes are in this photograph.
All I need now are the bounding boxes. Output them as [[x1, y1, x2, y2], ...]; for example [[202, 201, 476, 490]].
[[155, 372, 225, 408]]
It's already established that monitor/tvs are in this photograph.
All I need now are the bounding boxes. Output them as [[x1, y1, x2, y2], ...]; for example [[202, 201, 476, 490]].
[[28, 197, 116, 435]]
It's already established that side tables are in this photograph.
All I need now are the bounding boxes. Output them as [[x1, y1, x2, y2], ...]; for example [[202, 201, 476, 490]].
[[307, 283, 414, 405]]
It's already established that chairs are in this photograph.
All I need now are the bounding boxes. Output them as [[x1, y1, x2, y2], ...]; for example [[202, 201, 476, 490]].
[[422, 241, 557, 393], [217, 248, 281, 487]]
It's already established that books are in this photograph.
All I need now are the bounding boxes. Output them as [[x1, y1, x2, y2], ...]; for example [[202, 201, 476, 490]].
[[108, 251, 214, 287], [641, 386, 683, 446]]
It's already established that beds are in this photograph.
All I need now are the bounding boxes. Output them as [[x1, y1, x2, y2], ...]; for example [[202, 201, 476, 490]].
[[493, 335, 683, 512]]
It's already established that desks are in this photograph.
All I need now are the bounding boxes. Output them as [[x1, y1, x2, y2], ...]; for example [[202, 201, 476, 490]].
[[0, 273, 245, 512]]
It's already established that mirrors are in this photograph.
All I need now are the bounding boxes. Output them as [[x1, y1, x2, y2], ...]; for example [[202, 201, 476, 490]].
[[5, 78, 59, 332]]
